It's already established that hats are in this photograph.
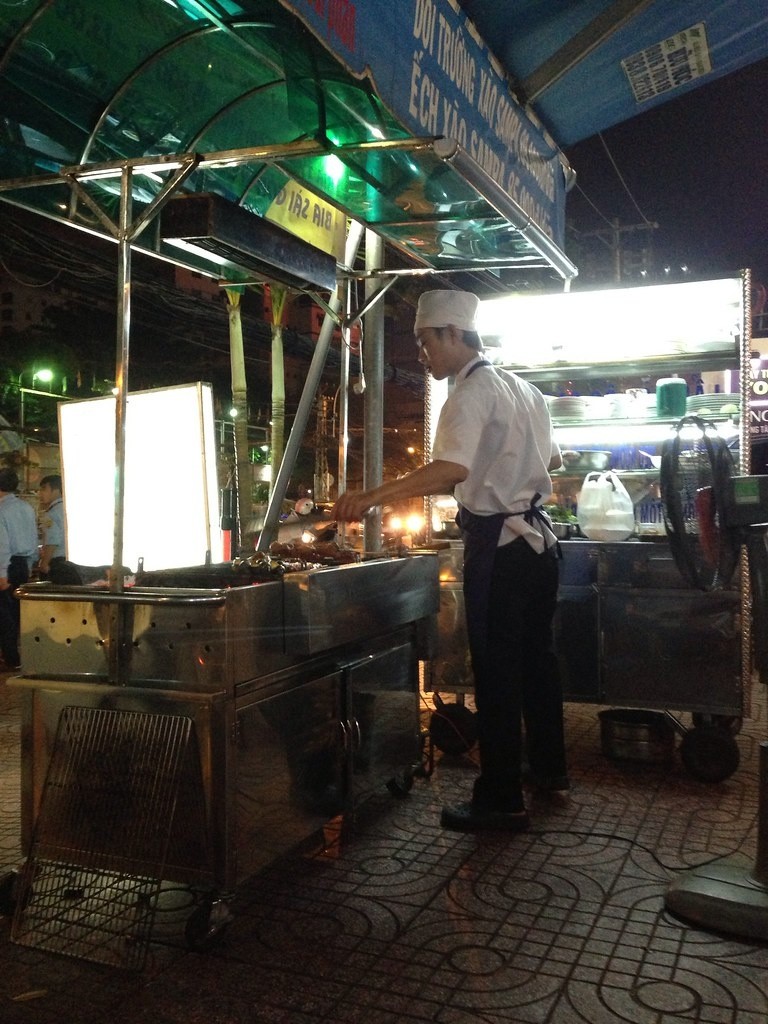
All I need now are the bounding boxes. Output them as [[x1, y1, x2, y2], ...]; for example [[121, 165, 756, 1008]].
[[413, 290, 480, 332]]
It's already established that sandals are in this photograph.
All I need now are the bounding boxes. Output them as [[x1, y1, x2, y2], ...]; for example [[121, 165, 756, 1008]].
[[520, 761, 570, 789], [441, 799, 533, 830]]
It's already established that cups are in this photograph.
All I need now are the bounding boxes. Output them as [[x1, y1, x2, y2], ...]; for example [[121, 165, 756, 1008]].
[[626, 387, 647, 418]]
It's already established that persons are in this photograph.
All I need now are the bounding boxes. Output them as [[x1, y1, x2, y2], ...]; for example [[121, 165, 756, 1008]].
[[0, 468, 38, 664], [329, 289, 563, 831], [39, 475, 67, 581]]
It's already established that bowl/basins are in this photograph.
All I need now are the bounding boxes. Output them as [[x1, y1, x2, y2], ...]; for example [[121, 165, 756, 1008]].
[[441, 519, 461, 539], [552, 522, 573, 540], [573, 524, 587, 538], [597, 709, 666, 745], [561, 450, 612, 469]]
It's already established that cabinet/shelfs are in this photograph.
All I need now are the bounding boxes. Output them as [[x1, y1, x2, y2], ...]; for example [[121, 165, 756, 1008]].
[[412, 273, 747, 775]]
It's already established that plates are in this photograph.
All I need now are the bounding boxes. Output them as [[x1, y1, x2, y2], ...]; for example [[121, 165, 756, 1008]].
[[647, 394, 657, 416], [687, 393, 741, 415], [544, 392, 626, 422]]
